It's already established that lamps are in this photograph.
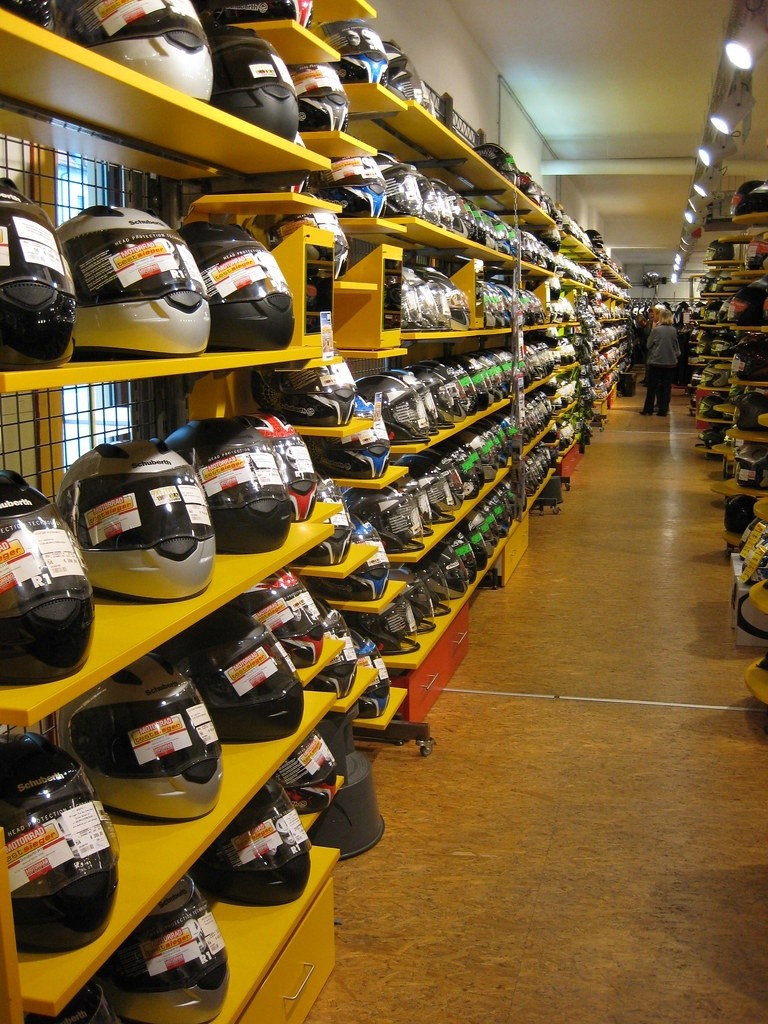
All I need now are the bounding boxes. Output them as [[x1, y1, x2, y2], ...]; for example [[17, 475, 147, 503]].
[[669, 13, 768, 284]]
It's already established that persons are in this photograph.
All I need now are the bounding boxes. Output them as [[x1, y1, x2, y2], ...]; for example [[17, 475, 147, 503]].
[[635, 304, 681, 417]]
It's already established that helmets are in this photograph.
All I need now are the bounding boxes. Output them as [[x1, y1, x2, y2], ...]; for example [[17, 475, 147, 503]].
[[0, 1, 638, 1023], [684, 180, 768, 535]]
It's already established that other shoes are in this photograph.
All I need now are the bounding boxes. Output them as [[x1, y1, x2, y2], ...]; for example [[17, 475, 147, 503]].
[[656, 410, 667, 416], [639, 409, 653, 416]]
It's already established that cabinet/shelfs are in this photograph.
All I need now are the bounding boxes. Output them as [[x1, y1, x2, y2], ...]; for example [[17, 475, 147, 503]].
[[666, 211, 767, 706], [1, 2, 631, 1024]]
